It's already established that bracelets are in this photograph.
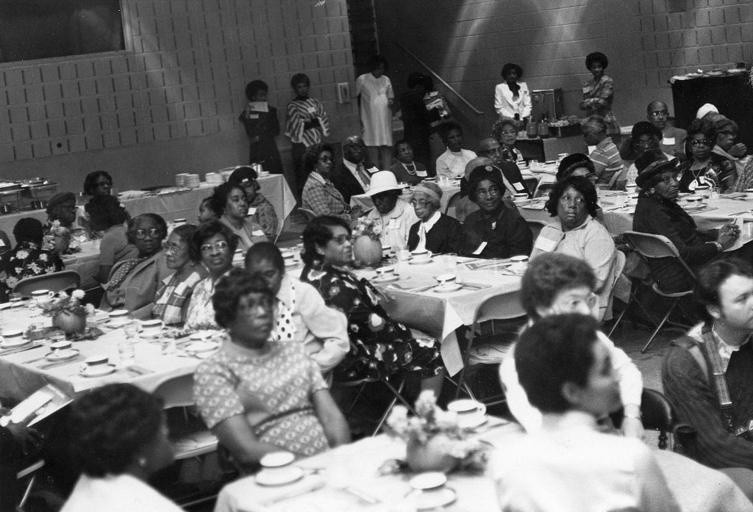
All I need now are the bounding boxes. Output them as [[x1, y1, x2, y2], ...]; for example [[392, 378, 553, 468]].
[[625, 415, 640, 419]]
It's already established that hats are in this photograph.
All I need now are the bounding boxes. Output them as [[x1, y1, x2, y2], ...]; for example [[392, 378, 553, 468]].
[[360, 171, 409, 198], [634, 150, 677, 189], [228, 167, 257, 184], [469, 165, 505, 196], [556, 153, 595, 179], [46, 192, 75, 208], [416, 181, 442, 201]]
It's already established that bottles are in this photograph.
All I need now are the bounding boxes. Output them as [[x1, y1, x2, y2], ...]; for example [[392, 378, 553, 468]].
[[527, 122, 549, 138]]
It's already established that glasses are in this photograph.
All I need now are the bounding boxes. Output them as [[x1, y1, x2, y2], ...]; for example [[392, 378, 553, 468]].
[[475, 187, 498, 195], [649, 109, 668, 116], [331, 233, 352, 244], [239, 297, 275, 314], [200, 241, 229, 253], [558, 293, 599, 309], [690, 138, 711, 146], [411, 199, 432, 207], [132, 226, 161, 241], [317, 156, 332, 162]]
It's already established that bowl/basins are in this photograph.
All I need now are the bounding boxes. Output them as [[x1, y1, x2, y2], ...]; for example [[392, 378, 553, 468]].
[[549, 126, 569, 138]]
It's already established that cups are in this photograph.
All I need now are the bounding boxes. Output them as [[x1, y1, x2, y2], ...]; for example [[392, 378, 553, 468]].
[[281, 244, 529, 281], [514, 160, 556, 201], [260, 398, 487, 502], [625, 183, 709, 207], [1, 289, 215, 373]]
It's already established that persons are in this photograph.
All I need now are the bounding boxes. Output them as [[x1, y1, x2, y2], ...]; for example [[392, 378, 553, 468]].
[[499, 253, 646, 442], [0, 402, 48, 512], [57, 383, 185, 512], [1, 52, 751, 403], [484, 313, 678, 512], [661, 260, 752, 470], [192, 267, 351, 470]]
